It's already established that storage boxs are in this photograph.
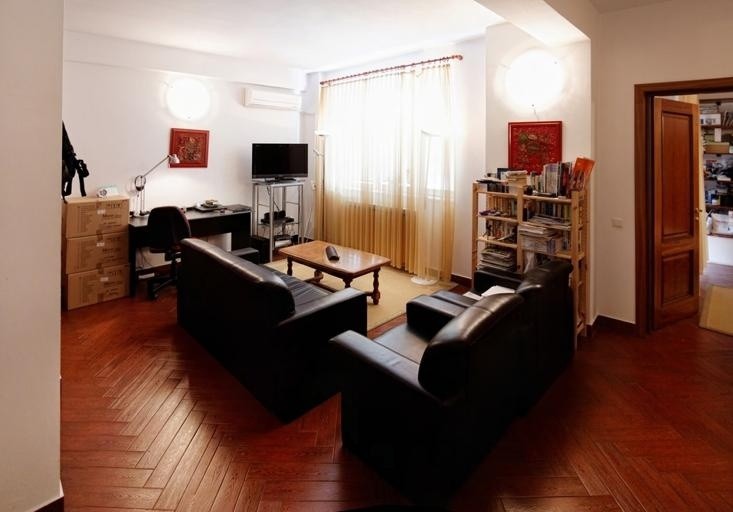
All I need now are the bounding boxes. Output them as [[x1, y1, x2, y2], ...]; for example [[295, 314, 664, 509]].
[[60, 195, 131, 311]]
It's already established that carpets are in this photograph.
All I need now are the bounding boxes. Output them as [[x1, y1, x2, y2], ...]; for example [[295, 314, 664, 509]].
[[262, 257, 459, 334], [698, 281, 733, 337]]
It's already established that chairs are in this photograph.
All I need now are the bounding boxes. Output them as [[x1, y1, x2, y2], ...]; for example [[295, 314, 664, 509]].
[[145, 206, 191, 299]]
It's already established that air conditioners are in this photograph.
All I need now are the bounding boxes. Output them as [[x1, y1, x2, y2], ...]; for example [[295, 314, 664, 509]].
[[242, 87, 302, 112]]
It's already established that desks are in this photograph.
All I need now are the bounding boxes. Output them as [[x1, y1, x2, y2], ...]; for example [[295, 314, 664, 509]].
[[127, 205, 251, 299]]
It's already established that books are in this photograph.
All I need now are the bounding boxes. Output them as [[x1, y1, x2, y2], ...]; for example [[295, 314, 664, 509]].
[[477, 156, 593, 330]]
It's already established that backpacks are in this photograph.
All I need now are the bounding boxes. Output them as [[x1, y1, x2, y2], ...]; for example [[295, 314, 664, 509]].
[[62, 121, 88, 204]]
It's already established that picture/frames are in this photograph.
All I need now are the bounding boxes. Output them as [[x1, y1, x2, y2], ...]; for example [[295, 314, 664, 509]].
[[169, 127, 209, 169], [506, 121, 562, 176]]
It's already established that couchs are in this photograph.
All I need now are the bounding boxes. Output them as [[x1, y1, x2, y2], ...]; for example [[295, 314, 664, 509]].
[[174, 237, 368, 424]]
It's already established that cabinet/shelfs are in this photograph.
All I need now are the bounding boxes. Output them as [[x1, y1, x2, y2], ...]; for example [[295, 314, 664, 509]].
[[695, 99, 733, 239], [251, 180, 304, 263], [471, 181, 590, 350]]
